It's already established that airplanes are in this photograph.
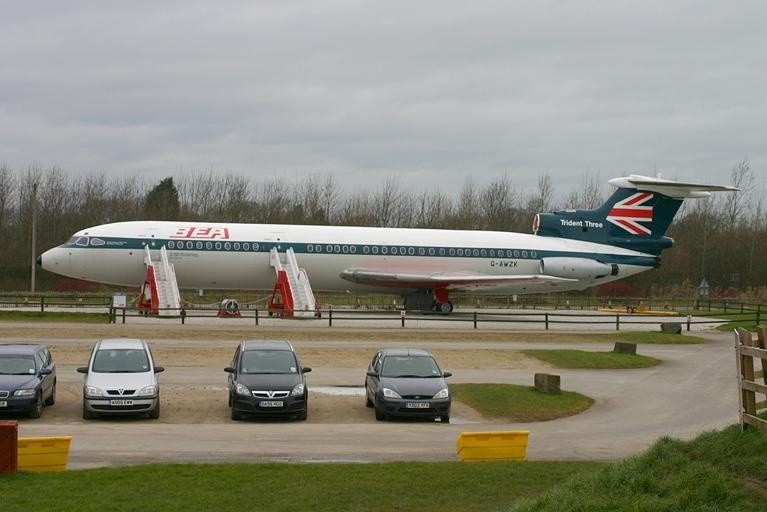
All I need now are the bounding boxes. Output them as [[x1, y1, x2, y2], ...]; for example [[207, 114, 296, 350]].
[[37, 173, 741, 315]]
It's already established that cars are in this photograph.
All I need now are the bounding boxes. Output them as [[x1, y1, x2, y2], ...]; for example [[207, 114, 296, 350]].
[[224, 335, 311, 420], [365, 347, 451, 423], [77, 338, 164, 419], [0, 342, 56, 418]]
